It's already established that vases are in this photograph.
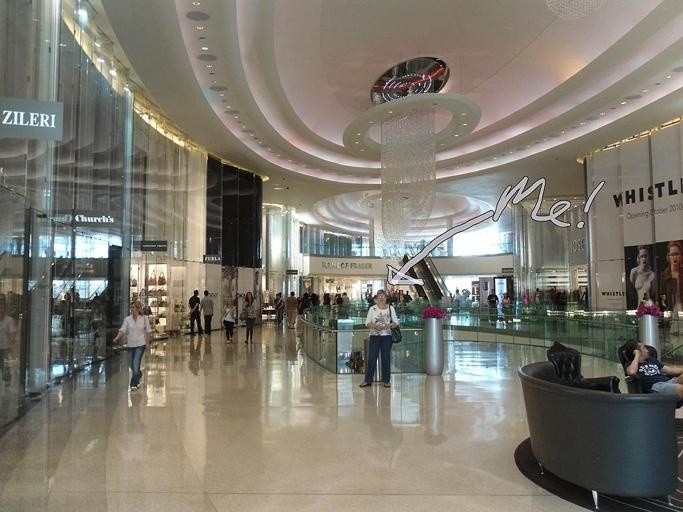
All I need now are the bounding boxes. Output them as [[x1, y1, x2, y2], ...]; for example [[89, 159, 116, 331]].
[[637, 315, 663, 364], [423, 315, 445, 377]]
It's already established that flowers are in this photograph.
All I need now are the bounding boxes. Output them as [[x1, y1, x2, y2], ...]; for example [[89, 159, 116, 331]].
[[636, 301, 663, 319], [421, 304, 447, 322]]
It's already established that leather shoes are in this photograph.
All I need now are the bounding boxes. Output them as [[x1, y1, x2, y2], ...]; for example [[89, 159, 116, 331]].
[[360, 382, 371, 387], [384, 383, 391, 387]]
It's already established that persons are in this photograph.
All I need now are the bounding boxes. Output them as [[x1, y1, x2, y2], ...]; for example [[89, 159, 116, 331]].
[[240, 344, 253, 373], [359, 289, 398, 387], [202, 336, 213, 376], [660, 241, 683, 312], [188, 291, 412, 343], [220, 344, 234, 376], [188, 344, 201, 375], [0, 301, 16, 387], [487, 287, 588, 326], [624, 339, 683, 401], [658, 293, 673, 311], [640, 292, 654, 308], [113, 301, 151, 390], [364, 387, 403, 472], [119, 390, 150, 460], [630, 245, 655, 309]]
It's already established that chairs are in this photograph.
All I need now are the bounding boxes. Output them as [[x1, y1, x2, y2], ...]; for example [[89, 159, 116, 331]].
[[544, 339, 624, 397], [618, 343, 683, 419]]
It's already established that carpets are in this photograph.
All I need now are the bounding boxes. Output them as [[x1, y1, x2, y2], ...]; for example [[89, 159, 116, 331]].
[[513, 408, 682, 511]]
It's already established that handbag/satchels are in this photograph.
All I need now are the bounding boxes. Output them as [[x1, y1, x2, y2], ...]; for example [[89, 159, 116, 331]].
[[390, 320, 402, 342]]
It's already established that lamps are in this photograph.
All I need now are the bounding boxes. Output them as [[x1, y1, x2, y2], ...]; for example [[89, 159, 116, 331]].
[[340, 55, 487, 241]]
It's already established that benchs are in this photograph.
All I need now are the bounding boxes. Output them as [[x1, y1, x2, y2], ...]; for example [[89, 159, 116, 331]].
[[511, 357, 681, 511]]
